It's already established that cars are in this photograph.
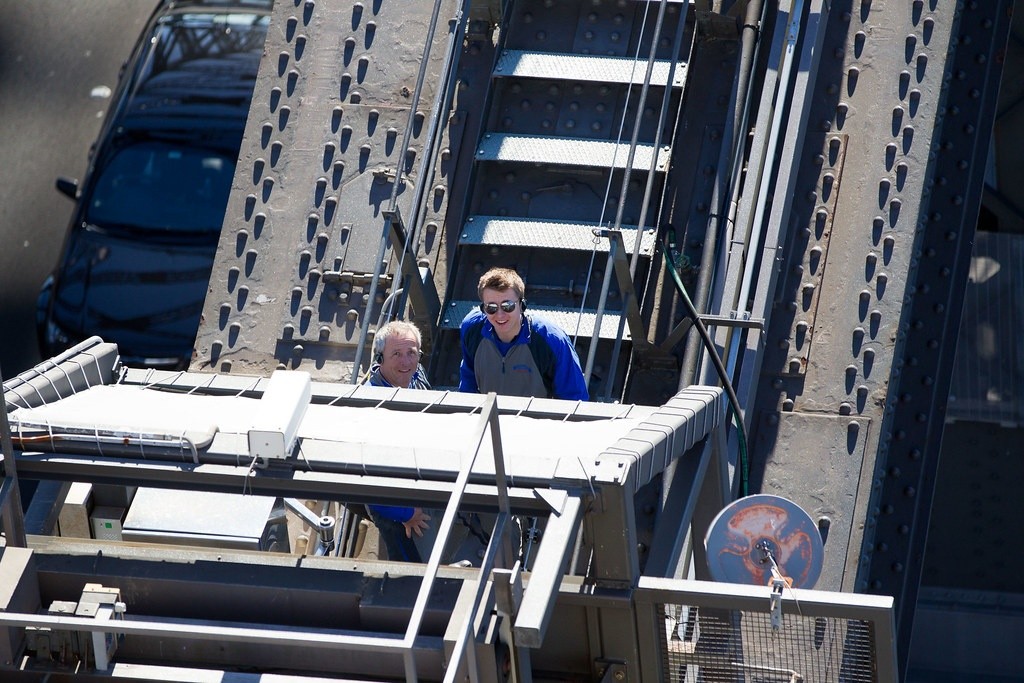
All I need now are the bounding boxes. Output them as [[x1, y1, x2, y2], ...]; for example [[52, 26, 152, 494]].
[[30, 1, 275, 372]]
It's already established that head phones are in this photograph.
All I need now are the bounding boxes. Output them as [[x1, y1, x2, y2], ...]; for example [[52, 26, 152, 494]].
[[374, 350, 423, 364], [480, 298, 526, 314]]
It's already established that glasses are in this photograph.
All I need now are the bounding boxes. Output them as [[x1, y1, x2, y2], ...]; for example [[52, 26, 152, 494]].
[[482, 300, 520, 315]]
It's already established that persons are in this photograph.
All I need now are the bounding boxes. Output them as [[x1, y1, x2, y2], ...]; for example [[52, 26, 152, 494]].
[[361, 321, 490, 564], [455, 265, 591, 402]]
